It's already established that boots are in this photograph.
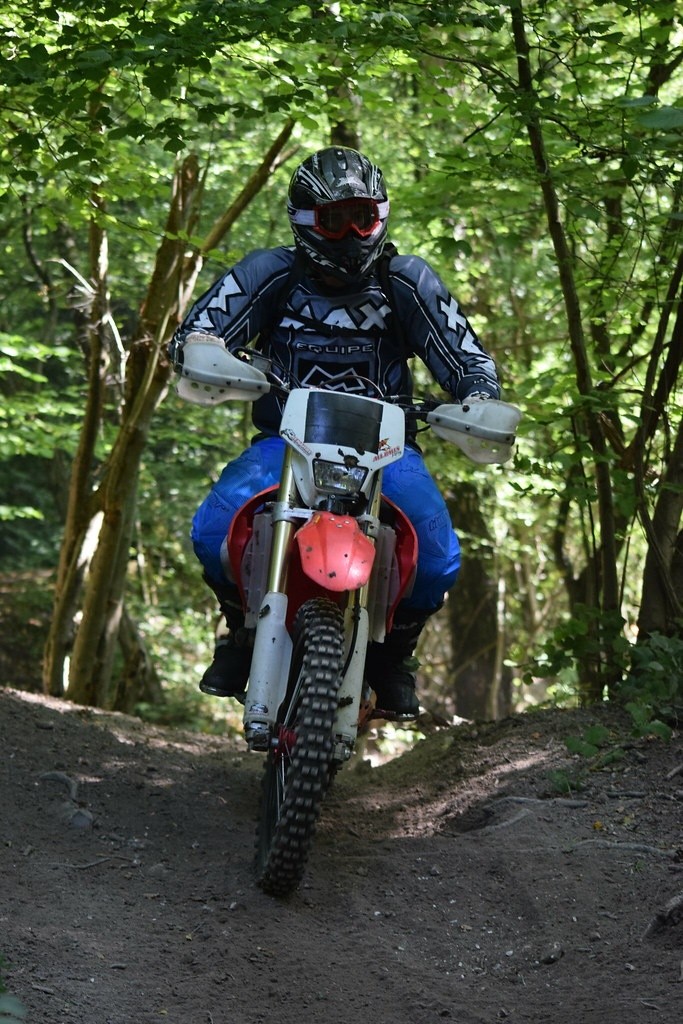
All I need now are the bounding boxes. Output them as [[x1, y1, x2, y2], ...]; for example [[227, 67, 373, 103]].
[[362, 599, 443, 714], [200, 570, 256, 697]]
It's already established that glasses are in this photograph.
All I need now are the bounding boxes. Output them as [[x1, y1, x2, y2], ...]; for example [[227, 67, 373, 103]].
[[310, 196, 381, 238]]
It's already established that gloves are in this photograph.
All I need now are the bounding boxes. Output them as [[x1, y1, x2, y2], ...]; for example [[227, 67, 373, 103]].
[[178, 331, 225, 351], [461, 390, 493, 407]]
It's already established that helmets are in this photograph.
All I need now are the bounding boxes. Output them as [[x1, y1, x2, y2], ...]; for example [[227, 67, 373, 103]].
[[289, 147, 389, 279]]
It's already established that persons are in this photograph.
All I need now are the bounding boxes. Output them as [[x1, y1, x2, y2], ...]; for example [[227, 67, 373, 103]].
[[167, 146, 500, 714]]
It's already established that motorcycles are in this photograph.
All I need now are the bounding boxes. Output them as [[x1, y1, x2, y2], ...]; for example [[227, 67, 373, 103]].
[[164, 325, 518, 899]]
